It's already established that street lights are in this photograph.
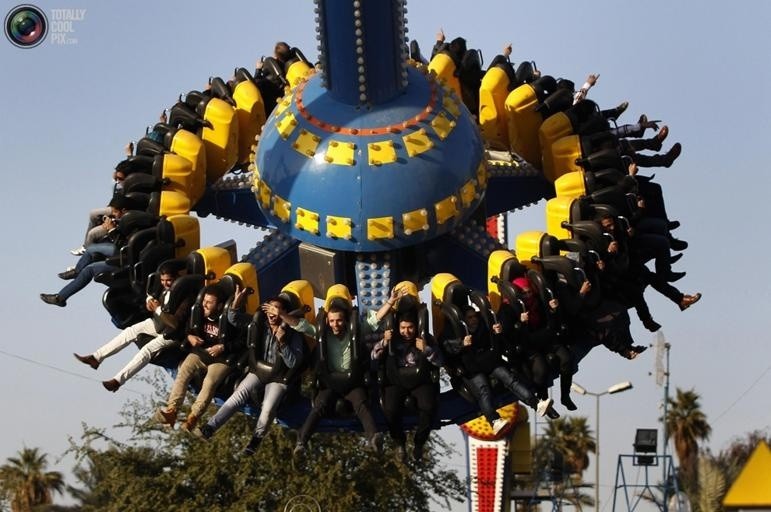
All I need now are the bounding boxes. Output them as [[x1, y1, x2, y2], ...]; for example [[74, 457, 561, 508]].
[[570, 379, 634, 512]]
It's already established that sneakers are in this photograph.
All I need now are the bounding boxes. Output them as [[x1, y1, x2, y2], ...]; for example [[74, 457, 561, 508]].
[[39, 293, 67, 307], [181, 417, 210, 442], [622, 344, 648, 360], [69, 247, 86, 256], [641, 318, 661, 332], [618, 101, 669, 152], [491, 418, 510, 435], [535, 398, 560, 419], [292, 442, 304, 457], [58, 265, 78, 279], [667, 219, 681, 232], [659, 236, 688, 283], [243, 433, 261, 457], [561, 395, 577, 411], [370, 433, 423, 463], [152, 409, 176, 430], [102, 379, 119, 393], [666, 142, 682, 168]]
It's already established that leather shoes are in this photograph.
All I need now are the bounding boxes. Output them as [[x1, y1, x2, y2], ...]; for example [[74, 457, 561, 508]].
[[73, 352, 99, 370], [679, 292, 701, 310]]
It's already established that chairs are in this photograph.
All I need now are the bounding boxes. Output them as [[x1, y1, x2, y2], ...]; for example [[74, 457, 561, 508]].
[[93, 35, 645, 432]]
